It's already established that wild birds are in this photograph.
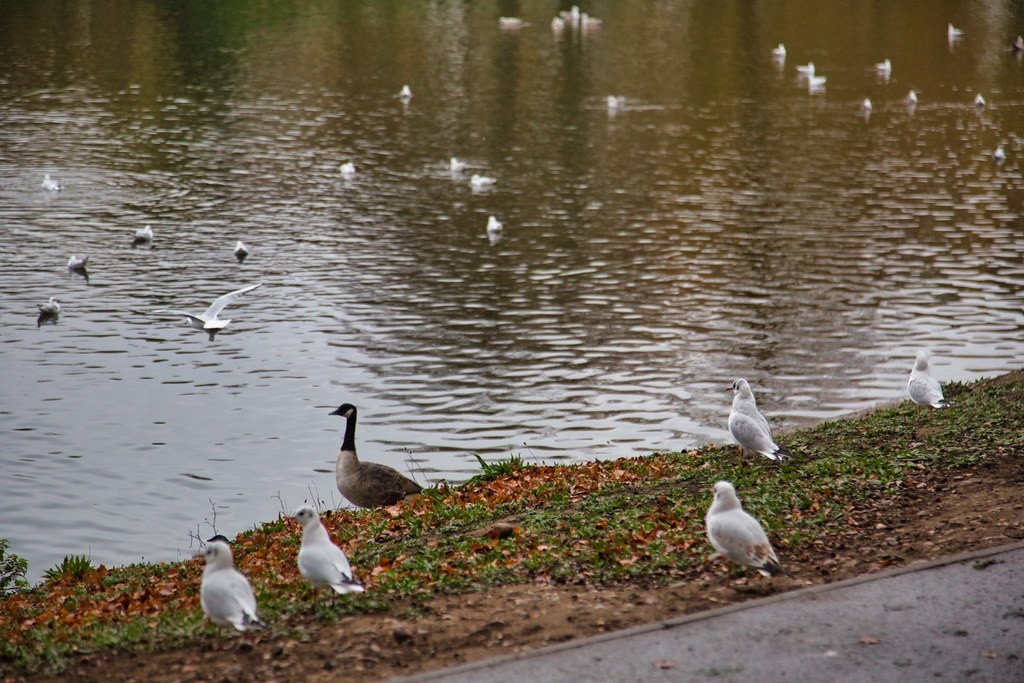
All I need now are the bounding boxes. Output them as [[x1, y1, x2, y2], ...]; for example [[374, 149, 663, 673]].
[[37, 296, 62, 313], [153, 283, 263, 342], [192, 541, 266, 632], [907, 90, 917, 103], [132, 224, 153, 240], [42, 173, 61, 191], [486, 214, 503, 232], [606, 95, 626, 107], [470, 173, 498, 186], [704, 481, 796, 584], [559, 5, 603, 26], [498, 16, 522, 25], [450, 157, 466, 172], [771, 43, 787, 57], [399, 84, 412, 98], [806, 73, 827, 86], [1013, 36, 1024, 51], [948, 23, 965, 37], [551, 16, 564, 27], [67, 256, 88, 268], [907, 350, 956, 422], [796, 61, 815, 73], [875, 58, 892, 72], [993, 147, 1007, 158], [234, 241, 248, 255], [290, 506, 365, 613], [340, 162, 355, 173], [328, 403, 424, 509], [726, 378, 799, 468], [861, 97, 872, 110], [973, 93, 985, 106]]
